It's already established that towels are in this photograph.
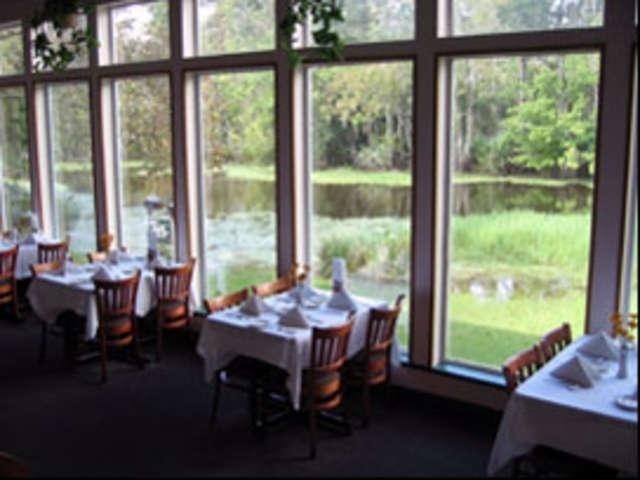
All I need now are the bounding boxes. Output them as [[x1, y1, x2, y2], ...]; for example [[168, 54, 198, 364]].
[[550, 352, 599, 390], [579, 330, 621, 361]]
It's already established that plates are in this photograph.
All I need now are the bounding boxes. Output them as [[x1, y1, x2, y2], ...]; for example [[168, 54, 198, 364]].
[[615, 393, 638, 411]]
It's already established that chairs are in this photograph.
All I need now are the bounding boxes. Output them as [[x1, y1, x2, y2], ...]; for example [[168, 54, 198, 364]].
[[498, 343, 544, 391], [534, 321, 573, 360]]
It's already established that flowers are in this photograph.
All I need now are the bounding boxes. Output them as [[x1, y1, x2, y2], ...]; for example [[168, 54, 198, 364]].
[[607, 309, 637, 344]]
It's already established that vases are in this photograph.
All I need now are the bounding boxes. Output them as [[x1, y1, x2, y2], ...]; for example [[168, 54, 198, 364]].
[[617, 344, 631, 380]]
